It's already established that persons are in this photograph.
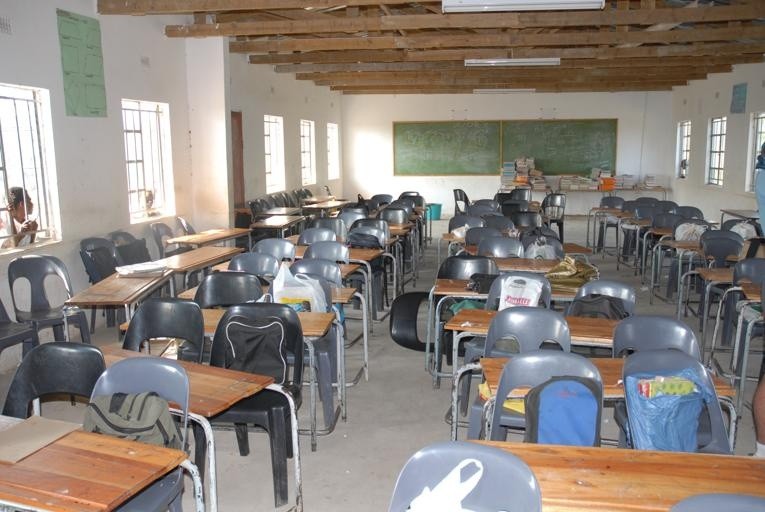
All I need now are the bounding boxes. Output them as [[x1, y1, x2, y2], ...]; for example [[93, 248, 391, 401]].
[[0, 186, 38, 248], [752, 142, 765, 456]]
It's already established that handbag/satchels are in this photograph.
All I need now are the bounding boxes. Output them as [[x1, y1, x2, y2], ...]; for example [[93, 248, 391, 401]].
[[544, 252, 599, 293], [139, 335, 200, 363], [465, 273, 500, 294]]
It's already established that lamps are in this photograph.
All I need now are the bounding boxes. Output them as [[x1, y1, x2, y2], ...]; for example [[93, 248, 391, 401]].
[[464, 58, 561, 67], [441, 0, 605, 13], [472, 89, 536, 93]]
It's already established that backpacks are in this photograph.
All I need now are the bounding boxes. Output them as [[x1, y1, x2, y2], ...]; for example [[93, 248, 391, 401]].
[[524, 374, 601, 446], [345, 233, 383, 266], [83, 391, 183, 452], [223, 315, 288, 384], [568, 294, 629, 357]]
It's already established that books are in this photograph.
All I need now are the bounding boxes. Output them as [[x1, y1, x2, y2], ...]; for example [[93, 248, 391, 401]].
[[559, 167, 664, 191], [499, 154, 549, 189], [116, 261, 168, 277]]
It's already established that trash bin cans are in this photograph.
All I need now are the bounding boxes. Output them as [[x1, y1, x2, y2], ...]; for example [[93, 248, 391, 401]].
[[425, 203, 441, 220]]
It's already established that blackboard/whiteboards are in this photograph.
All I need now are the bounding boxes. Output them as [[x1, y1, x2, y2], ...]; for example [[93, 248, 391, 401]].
[[393, 118, 618, 177]]
